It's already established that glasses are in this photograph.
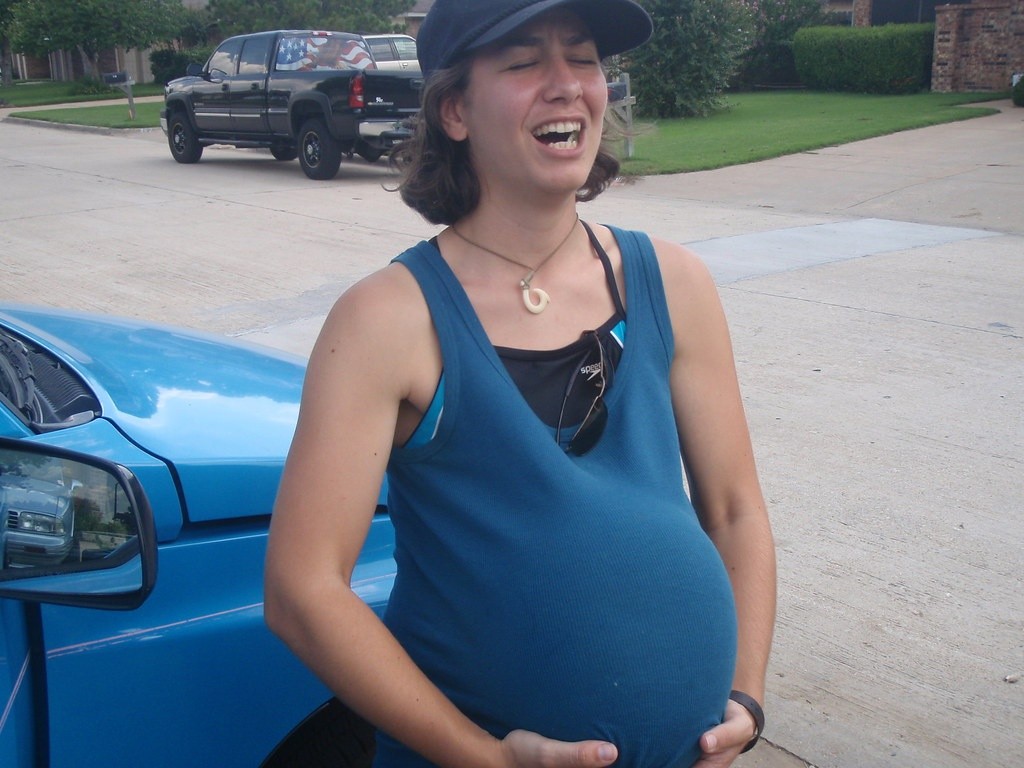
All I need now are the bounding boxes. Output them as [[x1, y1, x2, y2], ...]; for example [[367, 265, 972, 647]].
[[556, 330, 615, 456]]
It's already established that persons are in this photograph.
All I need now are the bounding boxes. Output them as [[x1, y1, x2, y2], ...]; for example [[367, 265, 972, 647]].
[[266, 0, 777, 768]]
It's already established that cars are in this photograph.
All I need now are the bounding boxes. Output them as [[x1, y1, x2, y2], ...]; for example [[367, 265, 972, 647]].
[[1, 301, 401, 768], [361, 33, 423, 72]]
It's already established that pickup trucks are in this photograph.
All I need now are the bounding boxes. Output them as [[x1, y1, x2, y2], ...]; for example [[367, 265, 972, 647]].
[[158, 29, 424, 180]]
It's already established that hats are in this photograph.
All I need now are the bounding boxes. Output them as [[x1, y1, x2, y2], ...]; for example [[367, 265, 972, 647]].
[[416, 0, 653, 84]]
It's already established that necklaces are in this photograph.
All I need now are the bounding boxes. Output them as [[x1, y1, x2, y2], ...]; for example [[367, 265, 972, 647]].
[[452, 213, 578, 313]]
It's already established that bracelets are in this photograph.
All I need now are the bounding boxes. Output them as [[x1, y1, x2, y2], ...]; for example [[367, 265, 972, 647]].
[[729, 686, 767, 755]]
